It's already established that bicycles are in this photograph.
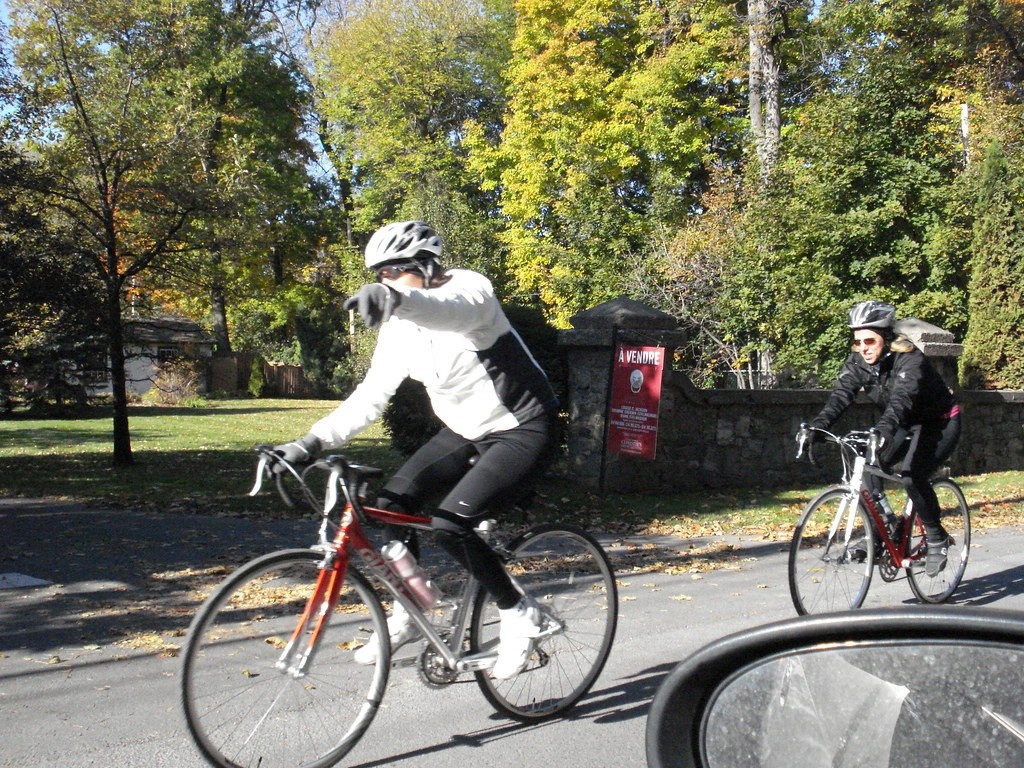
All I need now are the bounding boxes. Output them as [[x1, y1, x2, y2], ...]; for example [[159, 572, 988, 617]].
[[180, 444, 620, 768], [787, 422, 972, 616]]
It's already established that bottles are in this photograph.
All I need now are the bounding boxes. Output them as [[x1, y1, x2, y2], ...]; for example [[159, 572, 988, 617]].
[[877, 493, 898, 528], [383, 540, 444, 607]]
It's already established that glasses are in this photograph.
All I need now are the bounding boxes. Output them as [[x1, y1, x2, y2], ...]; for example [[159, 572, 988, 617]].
[[376, 261, 423, 283], [852, 338, 884, 346]]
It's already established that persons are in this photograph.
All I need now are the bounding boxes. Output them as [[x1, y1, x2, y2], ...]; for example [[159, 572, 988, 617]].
[[259, 221, 565, 682], [794, 300, 962, 577]]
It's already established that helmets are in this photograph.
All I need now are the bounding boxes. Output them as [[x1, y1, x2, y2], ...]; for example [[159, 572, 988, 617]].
[[848, 300, 896, 329], [365, 221, 442, 266]]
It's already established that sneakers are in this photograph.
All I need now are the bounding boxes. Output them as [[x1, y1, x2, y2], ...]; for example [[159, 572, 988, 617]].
[[925, 532, 950, 577], [354, 608, 423, 665], [843, 538, 881, 564], [493, 595, 542, 677]]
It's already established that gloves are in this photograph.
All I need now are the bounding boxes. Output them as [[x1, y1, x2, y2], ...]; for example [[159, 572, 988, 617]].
[[794, 422, 826, 451], [343, 283, 400, 329], [264, 434, 321, 481], [875, 420, 897, 465]]
